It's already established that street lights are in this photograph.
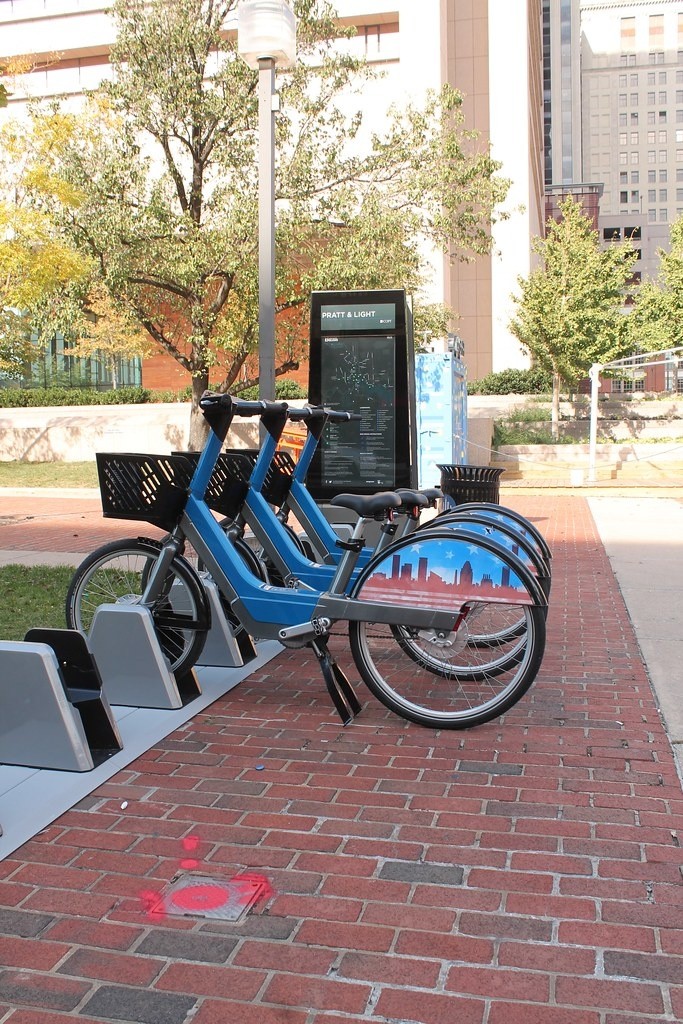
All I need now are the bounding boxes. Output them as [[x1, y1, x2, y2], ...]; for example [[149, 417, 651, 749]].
[[238, 2, 295, 454]]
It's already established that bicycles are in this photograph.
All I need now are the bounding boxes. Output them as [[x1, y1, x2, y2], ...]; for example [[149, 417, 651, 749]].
[[66, 389, 554, 732]]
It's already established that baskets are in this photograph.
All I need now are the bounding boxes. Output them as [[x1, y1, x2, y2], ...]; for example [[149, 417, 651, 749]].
[[171, 451, 254, 520], [95, 452, 194, 521], [226, 449, 296, 508]]
